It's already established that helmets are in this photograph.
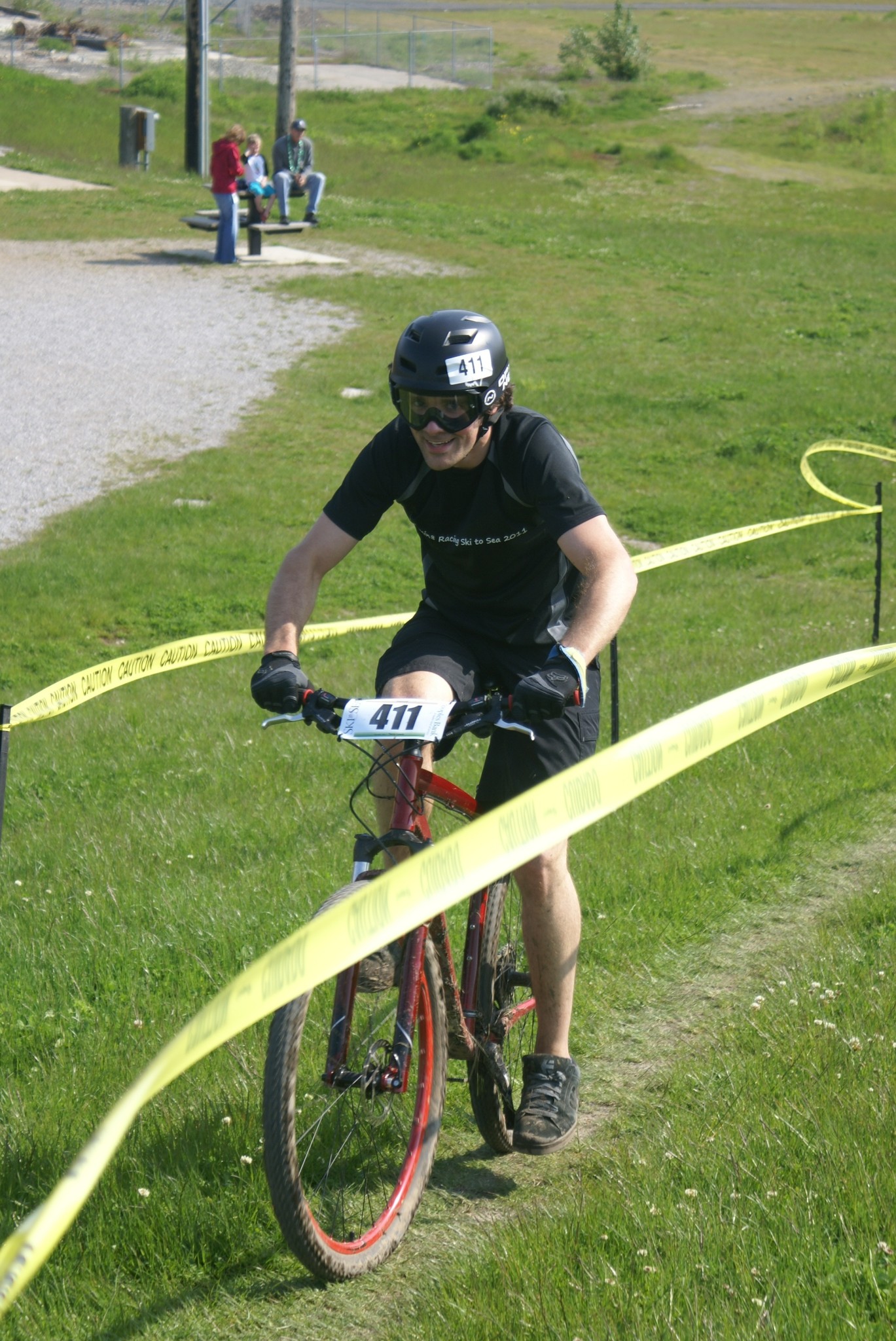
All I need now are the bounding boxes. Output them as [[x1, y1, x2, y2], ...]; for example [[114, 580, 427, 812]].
[[389, 309, 512, 420]]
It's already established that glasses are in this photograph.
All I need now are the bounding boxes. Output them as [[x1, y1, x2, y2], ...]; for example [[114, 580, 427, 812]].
[[390, 387, 483, 432]]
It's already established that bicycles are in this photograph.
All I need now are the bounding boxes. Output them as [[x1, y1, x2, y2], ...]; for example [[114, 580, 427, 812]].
[[260, 682, 590, 1282]]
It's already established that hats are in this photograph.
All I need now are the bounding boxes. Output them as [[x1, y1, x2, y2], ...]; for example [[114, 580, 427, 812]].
[[290, 119, 308, 131]]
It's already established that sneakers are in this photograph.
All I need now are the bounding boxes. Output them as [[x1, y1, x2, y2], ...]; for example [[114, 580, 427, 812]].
[[357, 936, 406, 992], [512, 1053, 580, 1156]]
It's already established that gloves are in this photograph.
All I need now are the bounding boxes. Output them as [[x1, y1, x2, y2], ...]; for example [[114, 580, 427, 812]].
[[250, 650, 315, 726], [512, 642, 587, 725]]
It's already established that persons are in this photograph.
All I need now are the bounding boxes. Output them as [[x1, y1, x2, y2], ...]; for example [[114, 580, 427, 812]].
[[272, 119, 328, 226], [241, 133, 276, 222], [210, 124, 245, 265], [250, 310, 638, 1155]]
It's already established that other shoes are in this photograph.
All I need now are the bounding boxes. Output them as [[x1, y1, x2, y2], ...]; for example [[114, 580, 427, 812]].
[[279, 217, 290, 225], [303, 214, 320, 223]]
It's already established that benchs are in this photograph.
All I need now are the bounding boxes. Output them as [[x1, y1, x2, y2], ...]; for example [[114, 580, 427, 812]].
[[248, 220, 319, 233], [195, 208, 249, 216], [179, 215, 218, 231]]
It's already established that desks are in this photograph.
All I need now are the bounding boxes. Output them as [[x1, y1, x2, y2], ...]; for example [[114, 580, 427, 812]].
[[204, 182, 306, 255]]
